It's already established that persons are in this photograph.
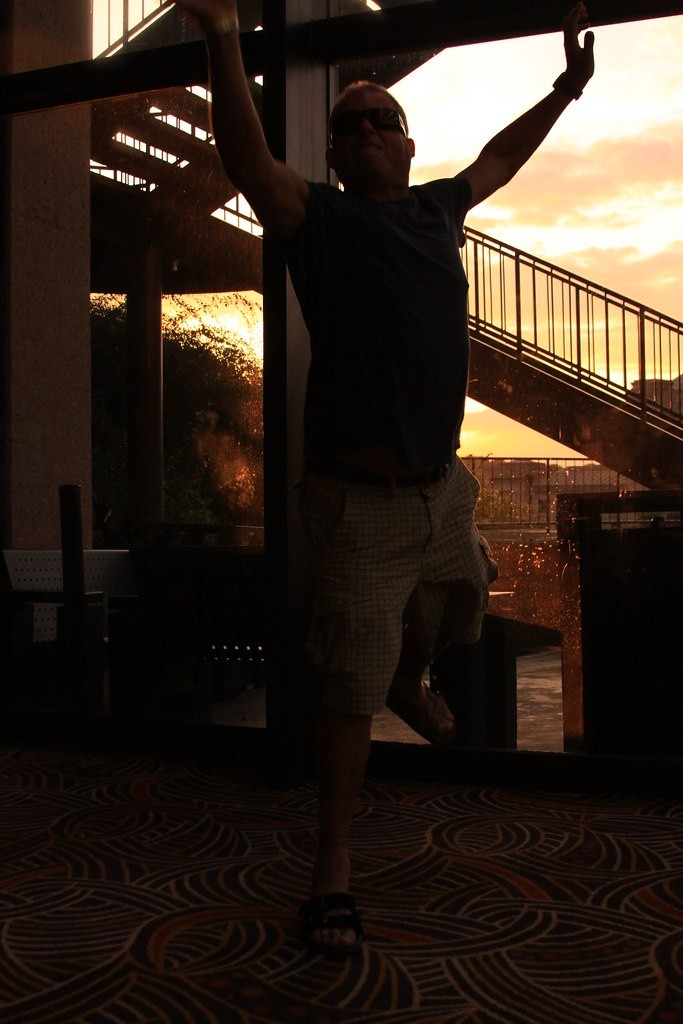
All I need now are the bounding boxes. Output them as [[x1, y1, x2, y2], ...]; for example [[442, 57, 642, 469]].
[[182, 0, 593, 954]]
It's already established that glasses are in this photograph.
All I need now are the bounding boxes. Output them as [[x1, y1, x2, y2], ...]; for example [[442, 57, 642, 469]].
[[329, 107, 407, 147]]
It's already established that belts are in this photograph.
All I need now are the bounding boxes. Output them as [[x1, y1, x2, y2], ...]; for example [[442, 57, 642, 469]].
[[308, 450, 455, 488]]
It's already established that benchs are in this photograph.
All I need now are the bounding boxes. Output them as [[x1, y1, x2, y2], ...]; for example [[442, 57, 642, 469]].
[[104, 543, 274, 707], [0, 549, 140, 694]]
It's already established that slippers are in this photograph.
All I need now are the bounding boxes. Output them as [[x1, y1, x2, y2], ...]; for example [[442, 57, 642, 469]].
[[384, 680, 457, 746], [297, 892, 364, 952]]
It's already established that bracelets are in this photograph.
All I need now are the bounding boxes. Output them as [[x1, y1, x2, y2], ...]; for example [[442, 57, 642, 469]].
[[553, 72, 582, 100]]
